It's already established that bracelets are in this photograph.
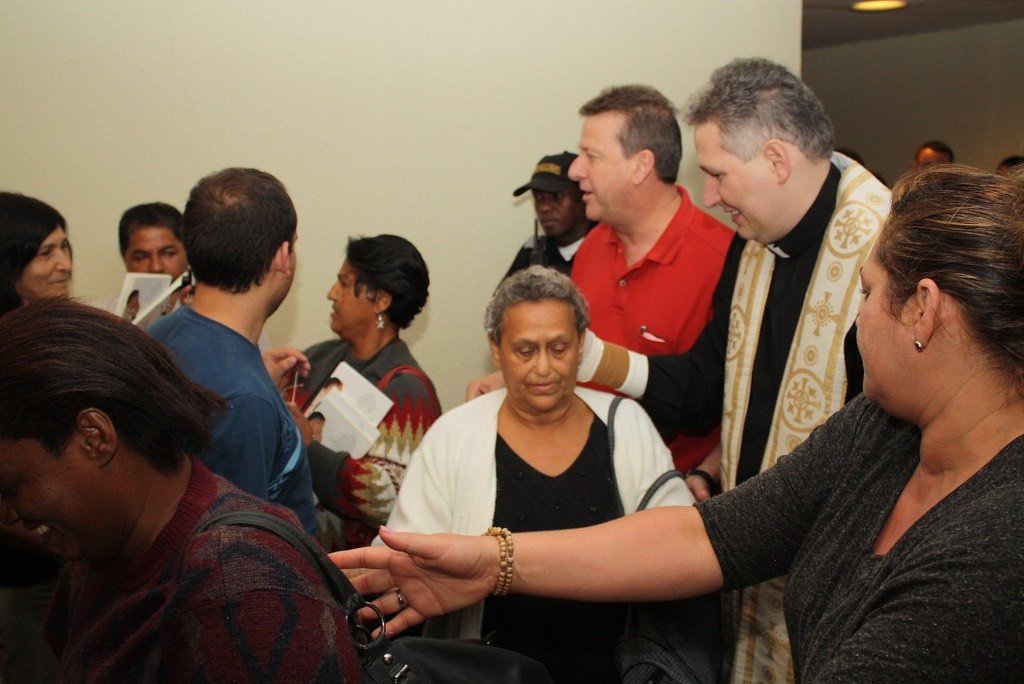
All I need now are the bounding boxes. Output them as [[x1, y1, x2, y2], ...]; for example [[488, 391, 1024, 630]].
[[686, 468, 715, 491], [485, 526, 514, 595]]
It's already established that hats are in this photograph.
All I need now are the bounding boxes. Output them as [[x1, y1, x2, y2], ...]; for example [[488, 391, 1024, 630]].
[[513, 152, 585, 199]]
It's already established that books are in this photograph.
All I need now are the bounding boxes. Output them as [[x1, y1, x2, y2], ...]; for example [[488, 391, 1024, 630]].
[[116, 271, 197, 331], [304, 361, 394, 460]]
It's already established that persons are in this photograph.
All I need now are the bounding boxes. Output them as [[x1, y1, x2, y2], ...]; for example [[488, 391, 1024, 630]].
[[121, 288, 141, 321], [572, 57, 894, 683], [328, 164, 1024, 684], [0, 191, 73, 684], [118, 202, 188, 284], [145, 166, 321, 539], [0, 297, 364, 684], [370, 264, 695, 684], [491, 151, 598, 302], [278, 233, 443, 549], [324, 377, 344, 396], [306, 409, 325, 442], [914, 141, 954, 170], [465, 84, 734, 503]]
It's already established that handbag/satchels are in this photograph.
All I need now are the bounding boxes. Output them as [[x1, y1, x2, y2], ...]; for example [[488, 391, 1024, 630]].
[[616, 598, 727, 684], [174, 512, 552, 684]]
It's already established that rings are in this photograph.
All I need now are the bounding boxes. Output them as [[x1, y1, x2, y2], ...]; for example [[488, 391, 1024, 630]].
[[397, 590, 405, 609]]
